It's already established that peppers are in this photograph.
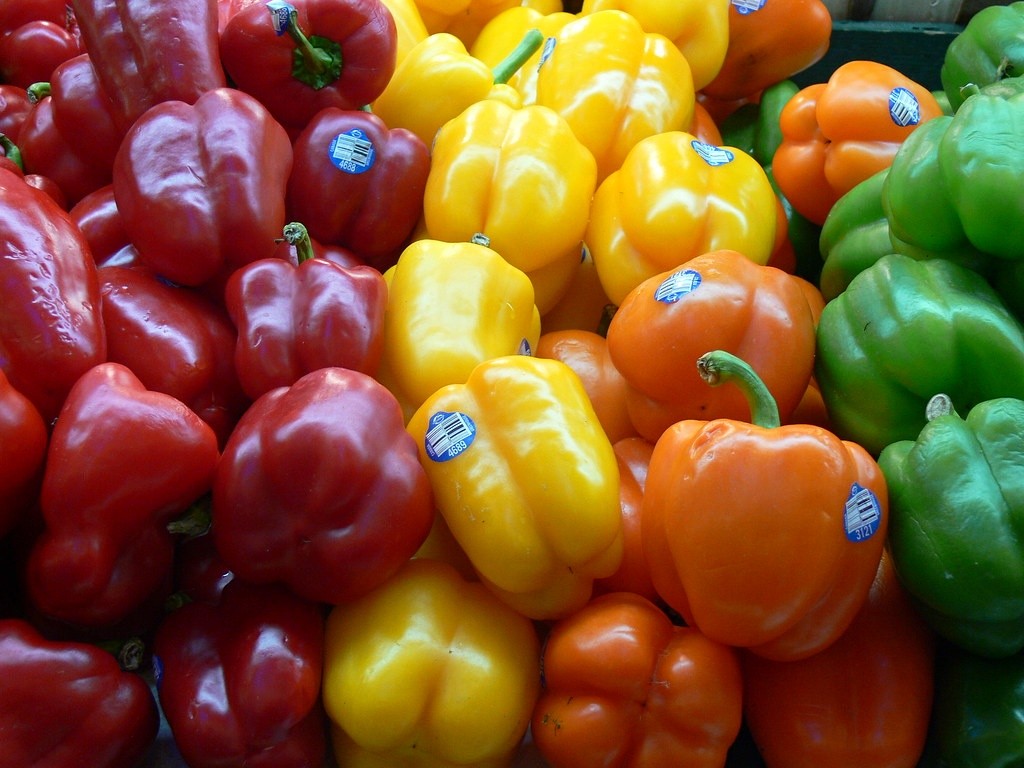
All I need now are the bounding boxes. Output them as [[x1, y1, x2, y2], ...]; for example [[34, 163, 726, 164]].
[[0, 0, 1024, 768]]
